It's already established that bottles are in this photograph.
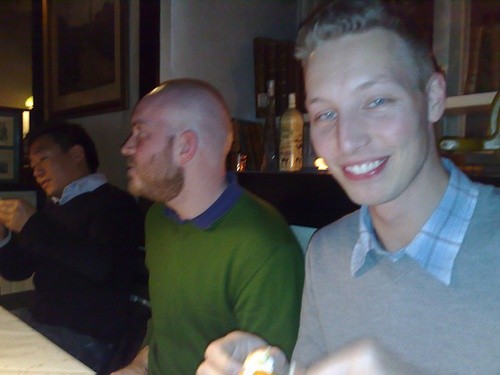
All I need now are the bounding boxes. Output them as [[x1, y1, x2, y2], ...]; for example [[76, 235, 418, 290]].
[[279, 94, 304, 172], [258, 80, 278, 172], [231, 121, 248, 172]]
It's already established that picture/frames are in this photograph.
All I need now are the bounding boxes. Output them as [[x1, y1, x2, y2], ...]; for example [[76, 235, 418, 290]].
[[41, 0, 130, 124], [0, 105, 23, 186]]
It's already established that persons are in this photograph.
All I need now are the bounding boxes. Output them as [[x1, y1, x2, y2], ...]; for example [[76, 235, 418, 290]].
[[0, 121, 152, 375], [194, 0, 500, 375], [107, 78, 308, 375]]
